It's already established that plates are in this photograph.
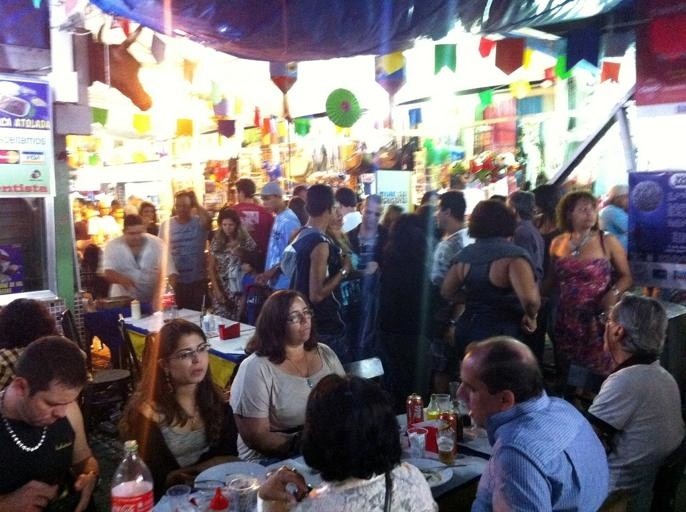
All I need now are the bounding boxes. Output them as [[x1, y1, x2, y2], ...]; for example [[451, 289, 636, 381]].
[[404, 458, 454, 486], [194, 461, 269, 506]]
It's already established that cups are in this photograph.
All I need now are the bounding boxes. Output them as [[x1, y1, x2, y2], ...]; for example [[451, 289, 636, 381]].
[[167, 483, 191, 512], [407, 394, 463, 465], [163, 304, 177, 322], [228, 478, 259, 512], [448, 382, 462, 414]]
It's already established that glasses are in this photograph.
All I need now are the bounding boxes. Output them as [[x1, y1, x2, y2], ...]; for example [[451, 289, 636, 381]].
[[287, 309, 313, 323], [598, 311, 617, 325], [166, 342, 210, 360]]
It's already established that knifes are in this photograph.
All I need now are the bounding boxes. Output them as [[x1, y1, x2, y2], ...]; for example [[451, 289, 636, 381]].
[[434, 463, 467, 468]]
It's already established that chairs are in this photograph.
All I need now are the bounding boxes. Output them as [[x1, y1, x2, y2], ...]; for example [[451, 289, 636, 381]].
[[647, 441, 684, 512], [593, 485, 640, 512], [340, 354, 386, 387], [54, 308, 132, 438]]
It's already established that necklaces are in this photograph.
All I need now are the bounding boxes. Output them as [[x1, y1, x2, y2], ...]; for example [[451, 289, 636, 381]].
[[0, 391, 48, 452]]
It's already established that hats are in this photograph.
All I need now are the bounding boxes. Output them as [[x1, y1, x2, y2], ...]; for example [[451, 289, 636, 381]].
[[253, 183, 282, 195], [608, 185, 628, 201]]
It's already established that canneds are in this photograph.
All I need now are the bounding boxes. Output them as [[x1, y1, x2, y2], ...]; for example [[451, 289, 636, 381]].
[[406, 394, 424, 430]]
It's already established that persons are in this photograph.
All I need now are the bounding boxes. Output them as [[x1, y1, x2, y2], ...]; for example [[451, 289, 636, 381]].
[[0, 298, 56, 389], [456, 336, 610, 512], [0, 335, 101, 512], [587, 293, 686, 511], [228, 290, 345, 467], [259, 374, 440, 512], [120, 320, 238, 506]]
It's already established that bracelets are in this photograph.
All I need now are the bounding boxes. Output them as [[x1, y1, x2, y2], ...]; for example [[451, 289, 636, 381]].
[[85, 470, 102, 491]]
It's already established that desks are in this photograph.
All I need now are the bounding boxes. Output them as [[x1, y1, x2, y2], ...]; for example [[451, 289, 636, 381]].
[[121, 304, 260, 396]]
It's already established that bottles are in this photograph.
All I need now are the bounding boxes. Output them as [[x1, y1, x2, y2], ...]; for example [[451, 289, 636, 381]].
[[203, 315, 215, 331], [131, 300, 141, 320], [161, 277, 178, 306], [109, 440, 153, 512]]
